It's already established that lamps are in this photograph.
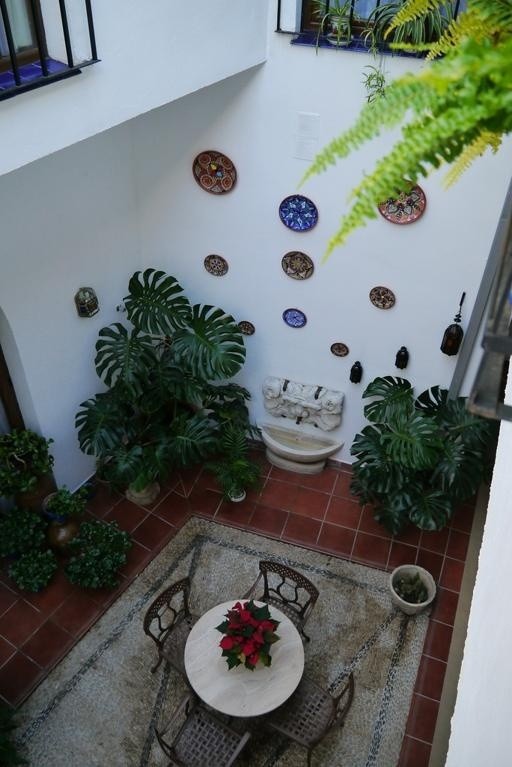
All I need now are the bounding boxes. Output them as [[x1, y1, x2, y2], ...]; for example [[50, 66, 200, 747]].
[[440, 289, 467, 356], [76, 286, 101, 318]]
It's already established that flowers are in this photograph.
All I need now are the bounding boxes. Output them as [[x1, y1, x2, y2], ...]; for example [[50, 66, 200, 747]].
[[218, 602, 275, 666]]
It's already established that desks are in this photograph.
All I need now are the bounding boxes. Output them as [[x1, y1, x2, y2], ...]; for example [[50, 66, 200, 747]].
[[184, 600, 305, 718]]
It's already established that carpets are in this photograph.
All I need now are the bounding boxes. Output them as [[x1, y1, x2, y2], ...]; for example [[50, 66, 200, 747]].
[[7, 515, 433, 766]]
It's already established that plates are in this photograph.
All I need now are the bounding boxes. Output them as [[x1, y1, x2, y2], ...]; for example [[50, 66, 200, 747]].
[[238, 320, 256, 336], [282, 307, 307, 329], [369, 285, 396, 311], [192, 150, 238, 195], [203, 254, 228, 277], [376, 178, 427, 225], [277, 194, 319, 233], [281, 251, 315, 280], [330, 342, 350, 358]]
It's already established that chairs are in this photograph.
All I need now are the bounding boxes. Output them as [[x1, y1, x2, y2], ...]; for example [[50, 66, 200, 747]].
[[142, 577, 204, 690], [239, 560, 319, 642], [257, 671, 354, 766], [153, 693, 254, 766]]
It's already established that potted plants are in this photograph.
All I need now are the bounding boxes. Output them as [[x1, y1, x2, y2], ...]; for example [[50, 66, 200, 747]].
[[389, 565, 436, 617], [40, 487, 96, 553], [302, 0, 361, 56]]
